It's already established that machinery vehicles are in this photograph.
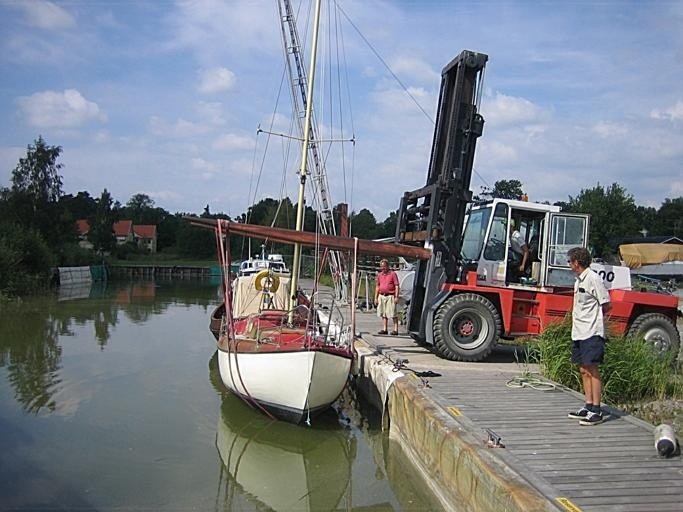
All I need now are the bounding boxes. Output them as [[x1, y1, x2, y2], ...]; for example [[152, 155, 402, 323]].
[[182, 50, 680, 370]]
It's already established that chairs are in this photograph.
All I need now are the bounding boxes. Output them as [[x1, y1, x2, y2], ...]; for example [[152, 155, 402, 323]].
[[510, 235, 543, 276]]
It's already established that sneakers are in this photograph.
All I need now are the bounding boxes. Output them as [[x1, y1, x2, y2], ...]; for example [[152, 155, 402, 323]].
[[568, 408, 605, 426], [378, 330, 398, 335]]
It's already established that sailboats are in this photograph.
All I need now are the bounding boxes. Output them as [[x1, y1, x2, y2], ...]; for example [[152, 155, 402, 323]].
[[215, 396, 351, 510]]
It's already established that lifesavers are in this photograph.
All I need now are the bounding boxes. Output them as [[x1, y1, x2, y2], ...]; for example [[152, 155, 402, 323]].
[[255, 269, 279, 292]]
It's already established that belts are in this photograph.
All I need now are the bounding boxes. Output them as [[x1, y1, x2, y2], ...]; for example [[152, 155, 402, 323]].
[[379, 293, 394, 296]]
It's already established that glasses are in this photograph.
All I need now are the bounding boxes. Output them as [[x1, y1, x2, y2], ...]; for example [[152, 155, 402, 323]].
[[567, 260, 570, 263]]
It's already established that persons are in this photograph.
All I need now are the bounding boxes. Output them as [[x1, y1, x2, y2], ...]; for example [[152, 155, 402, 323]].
[[372, 259, 400, 335], [565, 246, 614, 425], [502, 218, 529, 284]]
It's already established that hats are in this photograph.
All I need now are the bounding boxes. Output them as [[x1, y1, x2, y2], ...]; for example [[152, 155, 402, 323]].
[[501, 218, 515, 226]]
